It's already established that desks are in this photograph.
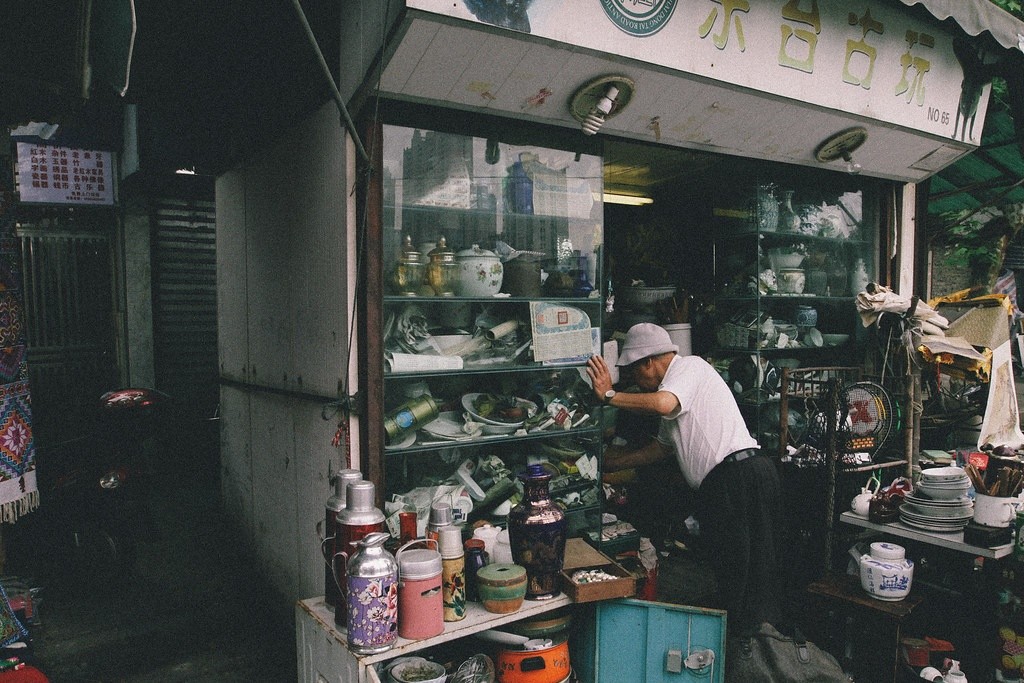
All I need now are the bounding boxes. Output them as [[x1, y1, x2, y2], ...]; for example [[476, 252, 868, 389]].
[[838, 508, 1016, 683]]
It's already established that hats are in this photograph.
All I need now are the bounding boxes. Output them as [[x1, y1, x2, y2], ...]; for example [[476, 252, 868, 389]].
[[614, 322, 679, 367]]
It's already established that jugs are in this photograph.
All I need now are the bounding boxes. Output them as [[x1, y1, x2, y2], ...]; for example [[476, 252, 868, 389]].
[[471, 524, 502, 564], [316, 469, 363, 612], [332, 532, 397, 654], [322, 480, 385, 634]]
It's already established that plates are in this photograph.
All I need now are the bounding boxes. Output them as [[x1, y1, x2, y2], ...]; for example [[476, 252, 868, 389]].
[[385, 432, 417, 448], [525, 639, 552, 650], [422, 394, 538, 440], [385, 657, 446, 683], [899, 495, 974, 530]]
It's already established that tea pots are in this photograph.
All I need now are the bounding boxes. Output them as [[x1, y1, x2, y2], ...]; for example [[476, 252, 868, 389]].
[[851, 477, 880, 516]]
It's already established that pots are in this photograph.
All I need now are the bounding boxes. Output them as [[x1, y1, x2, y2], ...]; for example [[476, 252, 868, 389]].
[[498, 633, 570, 683]]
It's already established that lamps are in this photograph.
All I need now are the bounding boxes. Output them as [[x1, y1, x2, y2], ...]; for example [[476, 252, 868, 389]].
[[603, 180, 655, 206], [579, 84, 619, 137]]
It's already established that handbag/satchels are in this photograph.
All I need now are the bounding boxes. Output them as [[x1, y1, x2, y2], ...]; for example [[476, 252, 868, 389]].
[[726, 622, 847, 683]]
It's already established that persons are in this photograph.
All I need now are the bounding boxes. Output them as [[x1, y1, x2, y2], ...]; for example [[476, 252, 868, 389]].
[[586, 323, 789, 636]]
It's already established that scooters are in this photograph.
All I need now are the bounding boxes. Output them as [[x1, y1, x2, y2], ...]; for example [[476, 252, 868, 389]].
[[47, 385, 173, 608]]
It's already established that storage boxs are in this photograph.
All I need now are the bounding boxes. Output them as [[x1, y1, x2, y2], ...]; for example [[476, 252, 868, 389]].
[[559, 550, 633, 604]]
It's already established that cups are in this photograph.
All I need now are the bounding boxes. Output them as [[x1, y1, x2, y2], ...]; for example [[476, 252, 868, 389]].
[[395, 538, 445, 641], [974, 491, 1014, 528], [384, 394, 439, 438]]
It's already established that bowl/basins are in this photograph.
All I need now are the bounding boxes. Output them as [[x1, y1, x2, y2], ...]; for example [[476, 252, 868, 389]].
[[804, 328, 823, 346], [916, 466, 972, 500], [623, 285, 677, 306], [823, 334, 850, 346]]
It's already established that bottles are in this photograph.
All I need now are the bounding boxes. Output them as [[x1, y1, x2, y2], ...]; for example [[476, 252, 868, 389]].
[[454, 490, 473, 514], [573, 257, 594, 298], [425, 503, 452, 550], [945, 670, 967, 683], [851, 258, 868, 296], [391, 513, 426, 555], [493, 530, 514, 565], [438, 526, 466, 621], [502, 162, 535, 215], [920, 667, 943, 682], [463, 539, 490, 601]]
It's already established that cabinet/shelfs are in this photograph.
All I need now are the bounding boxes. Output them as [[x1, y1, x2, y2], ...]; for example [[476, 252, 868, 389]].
[[380, 200, 601, 539], [776, 365, 917, 654], [711, 166, 880, 451], [295, 590, 728, 682]]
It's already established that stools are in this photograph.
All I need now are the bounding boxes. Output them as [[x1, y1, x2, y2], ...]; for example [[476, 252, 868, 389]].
[[805, 570, 926, 683]]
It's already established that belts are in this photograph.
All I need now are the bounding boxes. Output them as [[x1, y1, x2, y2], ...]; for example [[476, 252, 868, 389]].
[[725, 448, 760, 464]]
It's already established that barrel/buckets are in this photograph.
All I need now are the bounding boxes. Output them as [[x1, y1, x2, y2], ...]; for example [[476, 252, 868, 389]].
[[662, 323, 692, 357]]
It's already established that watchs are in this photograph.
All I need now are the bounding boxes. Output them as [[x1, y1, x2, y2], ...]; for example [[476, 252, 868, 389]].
[[605, 390, 616, 406]]
